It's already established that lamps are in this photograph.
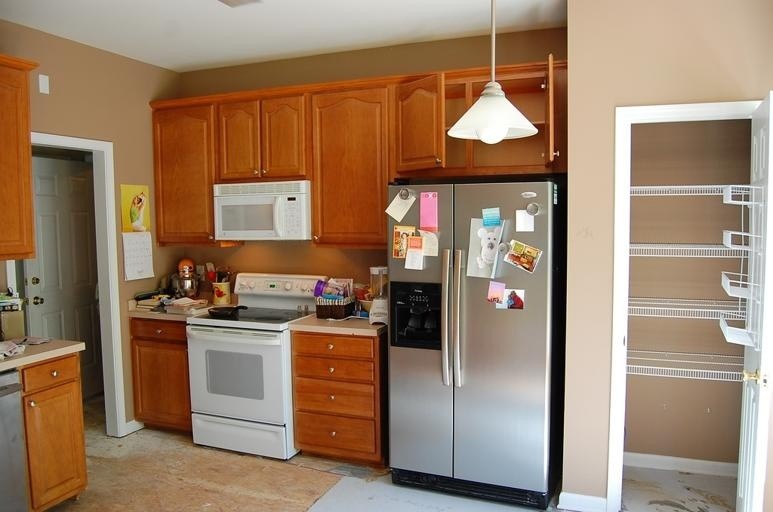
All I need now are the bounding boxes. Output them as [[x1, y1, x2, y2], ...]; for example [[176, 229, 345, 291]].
[[445, 0, 540, 144]]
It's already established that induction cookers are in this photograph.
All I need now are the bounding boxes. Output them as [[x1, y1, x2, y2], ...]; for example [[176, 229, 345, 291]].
[[186, 305, 316, 331]]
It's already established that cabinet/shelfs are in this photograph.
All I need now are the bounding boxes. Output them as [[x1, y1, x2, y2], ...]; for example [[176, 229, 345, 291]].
[[128, 317, 190, 435], [148, 94, 217, 247], [218, 82, 313, 182], [606, 89, 767, 512], [17, 352, 90, 511], [390, 53, 568, 180], [313, 78, 390, 249], [290, 331, 386, 469]]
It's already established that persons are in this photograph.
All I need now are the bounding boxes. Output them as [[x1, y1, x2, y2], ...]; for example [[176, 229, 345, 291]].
[[398, 233, 408, 257], [506, 295, 514, 308], [507, 291, 523, 309], [129, 192, 147, 231]]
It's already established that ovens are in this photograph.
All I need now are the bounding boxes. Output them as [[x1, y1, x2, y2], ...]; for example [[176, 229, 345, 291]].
[[185, 316, 299, 459]]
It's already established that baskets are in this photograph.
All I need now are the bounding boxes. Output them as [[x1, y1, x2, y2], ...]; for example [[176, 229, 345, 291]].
[[316, 302, 355, 319]]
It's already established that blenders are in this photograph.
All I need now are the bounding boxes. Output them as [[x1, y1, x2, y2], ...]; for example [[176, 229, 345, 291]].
[[368, 265, 388, 325]]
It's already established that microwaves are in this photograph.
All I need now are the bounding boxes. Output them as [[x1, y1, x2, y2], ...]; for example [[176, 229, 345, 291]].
[[212, 179, 311, 240]]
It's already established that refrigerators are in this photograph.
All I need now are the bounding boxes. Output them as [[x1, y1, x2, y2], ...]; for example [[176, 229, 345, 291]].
[[387, 175, 566, 512]]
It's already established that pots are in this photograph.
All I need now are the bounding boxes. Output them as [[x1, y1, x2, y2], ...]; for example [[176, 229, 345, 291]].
[[207, 306, 247, 318], [172, 270, 199, 296]]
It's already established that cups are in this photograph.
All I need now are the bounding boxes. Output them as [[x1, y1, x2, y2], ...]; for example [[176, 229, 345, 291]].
[[211, 282, 230, 305]]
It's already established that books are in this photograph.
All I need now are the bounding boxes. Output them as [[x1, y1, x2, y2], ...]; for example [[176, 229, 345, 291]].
[[0, 292, 23, 312], [10, 335, 52, 345], [136, 297, 208, 314]]
